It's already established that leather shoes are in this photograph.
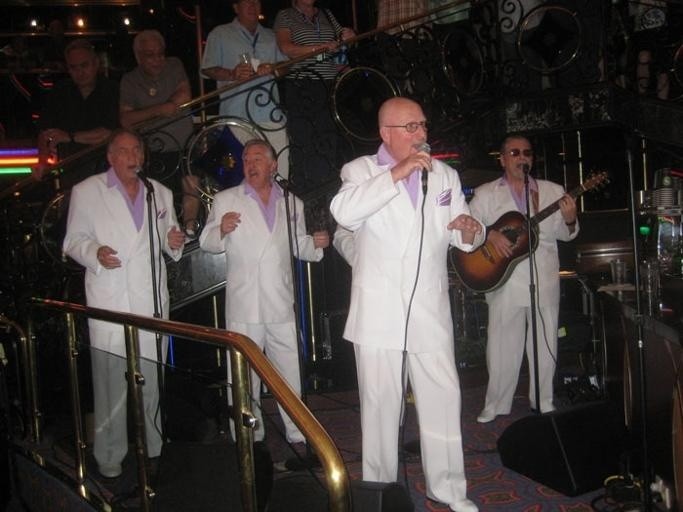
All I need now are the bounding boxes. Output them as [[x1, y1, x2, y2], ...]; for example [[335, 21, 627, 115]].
[[427, 494, 479, 512], [477, 411, 496, 423], [531, 405, 555, 413], [99, 464, 122, 477]]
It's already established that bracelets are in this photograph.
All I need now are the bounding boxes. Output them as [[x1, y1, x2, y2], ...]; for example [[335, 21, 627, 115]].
[[68, 130, 75, 144]]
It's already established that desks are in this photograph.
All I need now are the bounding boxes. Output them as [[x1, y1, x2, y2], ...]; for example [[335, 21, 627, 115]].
[[560, 236, 643, 427], [599, 286, 682, 512]]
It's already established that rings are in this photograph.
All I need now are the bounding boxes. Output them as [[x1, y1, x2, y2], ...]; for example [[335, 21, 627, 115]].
[[47, 136, 53, 142]]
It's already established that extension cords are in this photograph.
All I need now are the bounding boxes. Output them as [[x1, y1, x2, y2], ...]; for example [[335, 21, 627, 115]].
[[606, 478, 641, 502]]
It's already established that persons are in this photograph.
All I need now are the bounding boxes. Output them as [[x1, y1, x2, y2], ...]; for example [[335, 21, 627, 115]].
[[62, 128, 186, 503], [327, 97, 485, 512], [29, 36, 120, 242], [200, 0, 290, 184], [275, 0, 357, 197], [376, 0, 470, 60], [118, 28, 203, 247], [199, 139, 332, 466], [467, 130, 580, 424]]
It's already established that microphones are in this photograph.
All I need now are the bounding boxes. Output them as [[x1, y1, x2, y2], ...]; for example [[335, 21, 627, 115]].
[[622, 128, 633, 162], [521, 163, 530, 190], [419, 143, 432, 195], [267, 169, 298, 195], [132, 164, 155, 193]]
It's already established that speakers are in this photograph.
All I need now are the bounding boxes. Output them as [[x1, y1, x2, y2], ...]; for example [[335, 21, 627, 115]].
[[497, 401, 634, 497]]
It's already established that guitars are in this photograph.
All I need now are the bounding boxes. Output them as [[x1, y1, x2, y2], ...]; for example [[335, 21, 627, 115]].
[[450, 171, 609, 293]]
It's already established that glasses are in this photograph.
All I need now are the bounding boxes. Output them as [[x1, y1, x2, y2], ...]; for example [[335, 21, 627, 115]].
[[501, 149, 532, 157], [385, 122, 427, 133]]
